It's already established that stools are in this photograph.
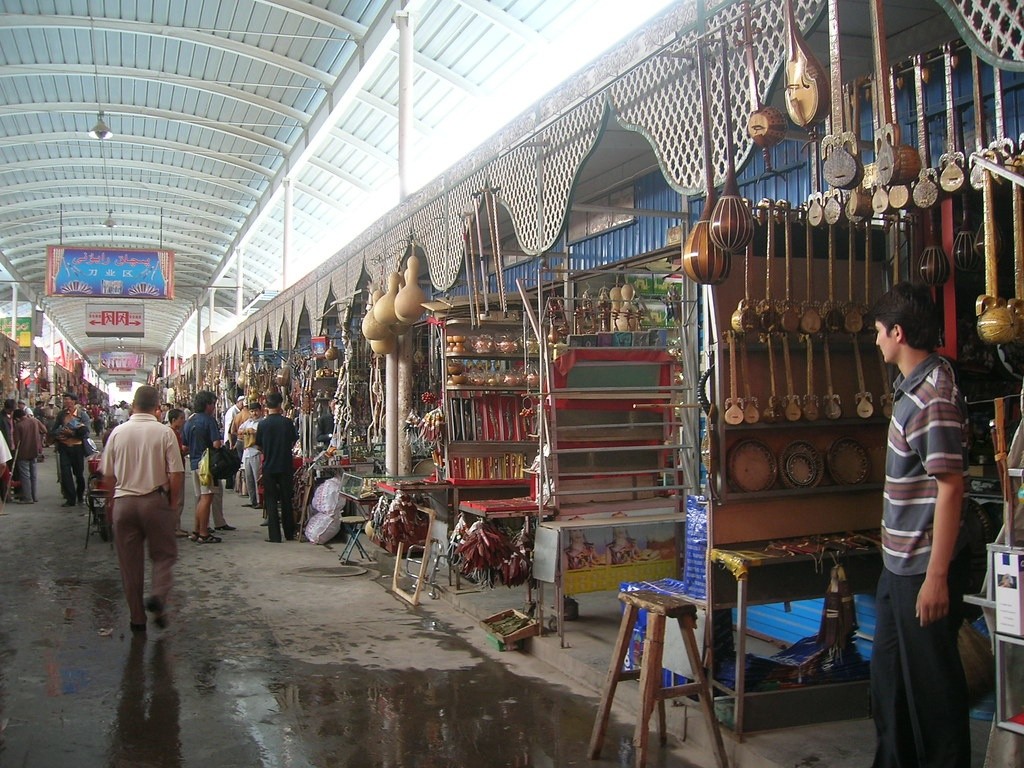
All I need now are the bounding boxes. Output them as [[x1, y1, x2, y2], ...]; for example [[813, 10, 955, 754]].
[[587, 589, 729, 768], [338, 517, 373, 564]]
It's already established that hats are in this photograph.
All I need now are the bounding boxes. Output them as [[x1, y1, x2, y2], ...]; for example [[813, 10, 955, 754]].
[[237, 396, 245, 402], [250, 403, 261, 409]]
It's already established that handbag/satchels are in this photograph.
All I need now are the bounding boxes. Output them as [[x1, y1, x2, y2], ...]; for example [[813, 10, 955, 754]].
[[209, 440, 241, 479], [83, 437, 97, 457], [243, 428, 256, 448]]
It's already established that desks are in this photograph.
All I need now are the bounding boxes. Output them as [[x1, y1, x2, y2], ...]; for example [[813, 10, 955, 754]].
[[532, 512, 687, 648], [457, 502, 554, 602], [703, 532, 889, 743]]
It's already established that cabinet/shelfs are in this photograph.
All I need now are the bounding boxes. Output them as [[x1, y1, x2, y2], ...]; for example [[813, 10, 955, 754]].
[[440, 318, 539, 391]]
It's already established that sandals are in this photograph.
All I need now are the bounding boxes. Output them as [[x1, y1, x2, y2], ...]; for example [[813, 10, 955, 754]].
[[198, 534, 222, 543], [191, 532, 200, 542]]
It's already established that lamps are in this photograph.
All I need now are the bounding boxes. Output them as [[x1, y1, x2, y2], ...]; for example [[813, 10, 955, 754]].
[[102, 214, 118, 229], [89, 111, 114, 140]]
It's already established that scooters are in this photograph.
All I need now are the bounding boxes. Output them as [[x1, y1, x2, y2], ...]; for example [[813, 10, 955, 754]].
[[86, 437, 113, 542]]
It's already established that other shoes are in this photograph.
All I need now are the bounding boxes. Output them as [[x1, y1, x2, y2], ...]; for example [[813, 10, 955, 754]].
[[215, 525, 236, 531], [1, 495, 13, 502], [254, 504, 264, 509], [175, 529, 188, 537], [207, 527, 215, 533], [146, 595, 164, 613], [264, 538, 281, 543], [32, 496, 39, 502], [286, 536, 293, 540], [16, 499, 33, 504], [77, 497, 84, 503], [130, 623, 146, 635], [241, 503, 252, 507], [62, 503, 73, 507]]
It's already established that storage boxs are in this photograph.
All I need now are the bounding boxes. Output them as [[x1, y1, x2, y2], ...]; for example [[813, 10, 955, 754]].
[[480, 607, 541, 645]]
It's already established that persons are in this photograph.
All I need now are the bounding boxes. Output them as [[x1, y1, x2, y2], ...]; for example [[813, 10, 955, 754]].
[[864, 280, 973, 768], [97, 386, 184, 632], [317, 398, 335, 450], [255, 393, 299, 542], [0, 393, 132, 507], [163, 390, 265, 542]]
